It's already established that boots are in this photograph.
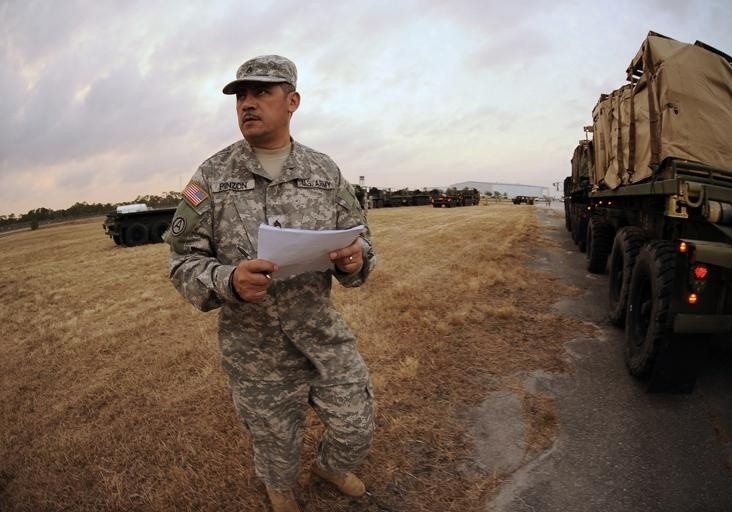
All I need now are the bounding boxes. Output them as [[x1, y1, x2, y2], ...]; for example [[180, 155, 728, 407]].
[[266, 488, 301, 512], [311, 459, 366, 497]]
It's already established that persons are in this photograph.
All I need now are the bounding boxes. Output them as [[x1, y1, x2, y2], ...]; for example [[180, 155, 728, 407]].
[[161, 56, 376, 512]]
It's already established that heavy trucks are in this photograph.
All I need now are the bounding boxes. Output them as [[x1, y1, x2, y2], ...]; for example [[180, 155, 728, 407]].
[[563, 31, 732, 395]]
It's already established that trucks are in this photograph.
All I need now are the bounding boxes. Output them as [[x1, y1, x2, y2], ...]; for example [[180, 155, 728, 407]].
[[102, 207, 177, 247], [352, 184, 480, 209]]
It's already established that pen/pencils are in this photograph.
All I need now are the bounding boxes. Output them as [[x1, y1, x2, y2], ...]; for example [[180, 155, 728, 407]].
[[237, 246, 272, 280]]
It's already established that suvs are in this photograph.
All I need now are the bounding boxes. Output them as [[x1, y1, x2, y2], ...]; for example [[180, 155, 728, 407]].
[[512, 196, 527, 204]]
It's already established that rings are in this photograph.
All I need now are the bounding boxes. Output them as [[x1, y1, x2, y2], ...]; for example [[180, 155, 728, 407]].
[[349, 256, 353, 261]]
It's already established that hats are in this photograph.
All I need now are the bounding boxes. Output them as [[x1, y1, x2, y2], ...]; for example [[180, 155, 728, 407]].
[[223, 55, 297, 94]]
